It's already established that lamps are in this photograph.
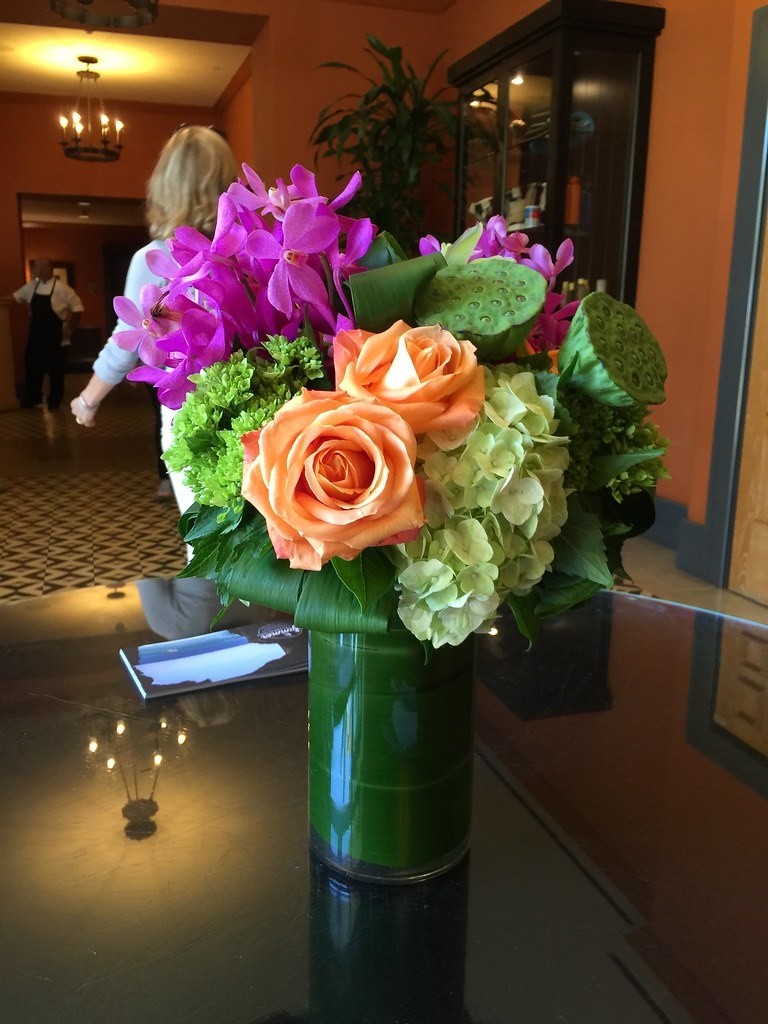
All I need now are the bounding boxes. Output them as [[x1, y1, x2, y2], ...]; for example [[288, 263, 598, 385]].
[[60, 52, 127, 164], [74, 701, 194, 847]]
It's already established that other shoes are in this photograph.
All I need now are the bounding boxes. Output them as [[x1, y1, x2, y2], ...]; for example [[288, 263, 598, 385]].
[[156, 478, 174, 498]]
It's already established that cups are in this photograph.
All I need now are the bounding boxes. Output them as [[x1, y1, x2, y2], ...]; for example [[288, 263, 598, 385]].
[[524, 205, 541, 228]]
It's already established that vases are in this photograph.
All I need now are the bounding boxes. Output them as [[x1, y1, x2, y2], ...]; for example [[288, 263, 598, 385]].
[[304, 622, 477, 889]]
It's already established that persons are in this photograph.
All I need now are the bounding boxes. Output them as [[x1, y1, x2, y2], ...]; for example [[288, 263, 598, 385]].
[[71, 123, 240, 563], [148, 383, 174, 505], [0, 257, 85, 413]]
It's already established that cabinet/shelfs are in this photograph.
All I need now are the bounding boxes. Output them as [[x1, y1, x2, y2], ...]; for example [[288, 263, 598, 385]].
[[446, 0, 666, 311]]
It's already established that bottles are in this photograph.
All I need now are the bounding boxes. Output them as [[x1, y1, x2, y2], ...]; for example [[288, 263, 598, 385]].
[[562, 175, 581, 226], [558, 278, 609, 309]]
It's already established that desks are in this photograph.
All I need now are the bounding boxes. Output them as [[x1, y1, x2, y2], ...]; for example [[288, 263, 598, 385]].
[[0, 580, 768, 1024]]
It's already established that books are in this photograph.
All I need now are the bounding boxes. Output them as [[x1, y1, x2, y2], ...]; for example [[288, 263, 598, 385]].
[[118, 619, 309, 701]]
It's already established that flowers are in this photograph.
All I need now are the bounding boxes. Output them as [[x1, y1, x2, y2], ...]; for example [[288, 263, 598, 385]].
[[121, 164, 671, 644]]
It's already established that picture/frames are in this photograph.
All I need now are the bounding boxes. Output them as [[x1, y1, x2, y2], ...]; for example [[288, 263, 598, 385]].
[[28, 258, 75, 289]]
